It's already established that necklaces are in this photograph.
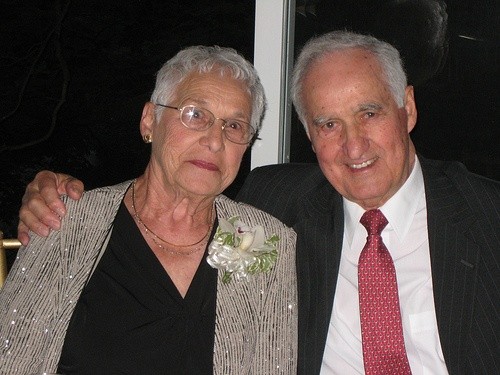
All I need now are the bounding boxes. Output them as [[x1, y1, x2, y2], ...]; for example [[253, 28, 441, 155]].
[[131, 180, 215, 256]]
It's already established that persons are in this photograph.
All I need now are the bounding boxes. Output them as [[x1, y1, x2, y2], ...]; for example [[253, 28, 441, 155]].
[[18, 29, 500, 375], [0, 45, 298, 375]]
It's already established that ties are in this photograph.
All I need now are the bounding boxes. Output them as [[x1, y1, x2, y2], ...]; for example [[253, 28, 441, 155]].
[[360, 209, 414, 372]]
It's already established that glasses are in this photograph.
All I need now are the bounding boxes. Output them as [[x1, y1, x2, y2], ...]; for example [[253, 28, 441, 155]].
[[157, 101, 257, 149]]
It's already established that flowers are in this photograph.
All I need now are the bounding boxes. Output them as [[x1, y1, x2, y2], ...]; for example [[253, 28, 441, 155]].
[[206, 216, 280, 282]]
